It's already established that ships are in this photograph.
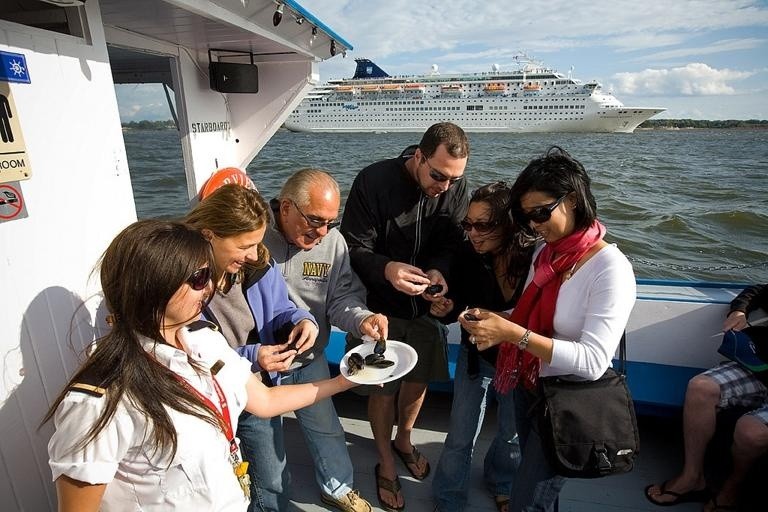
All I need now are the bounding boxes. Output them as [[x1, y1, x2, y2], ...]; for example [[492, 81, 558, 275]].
[[284, 51, 660, 134]]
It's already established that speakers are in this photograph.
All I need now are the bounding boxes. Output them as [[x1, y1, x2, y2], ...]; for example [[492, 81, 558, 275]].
[[207, 48, 258, 93]]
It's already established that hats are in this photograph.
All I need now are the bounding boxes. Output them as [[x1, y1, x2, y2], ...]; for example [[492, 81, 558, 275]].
[[723, 329, 768, 372]]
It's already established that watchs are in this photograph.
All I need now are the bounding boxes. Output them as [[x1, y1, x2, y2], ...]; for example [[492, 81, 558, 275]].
[[517, 329, 531, 351]]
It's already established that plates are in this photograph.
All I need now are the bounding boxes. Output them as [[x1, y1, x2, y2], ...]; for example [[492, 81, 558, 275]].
[[340, 339, 419, 386]]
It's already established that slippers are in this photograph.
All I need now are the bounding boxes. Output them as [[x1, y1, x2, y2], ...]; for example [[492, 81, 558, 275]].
[[392, 440, 430, 480], [645, 479, 731, 512], [494, 495, 510, 512], [375, 463, 405, 512]]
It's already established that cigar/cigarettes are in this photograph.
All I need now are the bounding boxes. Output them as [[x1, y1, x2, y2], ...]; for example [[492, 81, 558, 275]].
[[0, 199, 19, 205]]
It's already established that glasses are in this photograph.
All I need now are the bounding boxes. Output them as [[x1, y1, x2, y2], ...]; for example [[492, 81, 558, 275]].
[[422, 153, 463, 184], [185, 267, 210, 290], [461, 220, 499, 232], [522, 195, 566, 224], [293, 201, 340, 229]]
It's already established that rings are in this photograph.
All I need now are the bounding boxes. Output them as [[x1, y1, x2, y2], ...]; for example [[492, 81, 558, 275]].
[[470, 336, 476, 344]]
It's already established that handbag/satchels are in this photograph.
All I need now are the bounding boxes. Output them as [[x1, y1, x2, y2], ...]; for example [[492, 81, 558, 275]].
[[537, 368, 640, 478]]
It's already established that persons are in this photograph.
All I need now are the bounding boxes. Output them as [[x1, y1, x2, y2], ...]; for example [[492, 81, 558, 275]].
[[339, 122, 469, 512], [37, 219, 362, 512], [429, 180, 545, 512], [457, 145, 637, 512], [183, 183, 320, 512], [644, 282, 767, 512], [262, 167, 388, 512]]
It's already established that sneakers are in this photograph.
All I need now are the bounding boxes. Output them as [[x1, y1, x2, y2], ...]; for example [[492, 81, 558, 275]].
[[321, 489, 372, 512]]
